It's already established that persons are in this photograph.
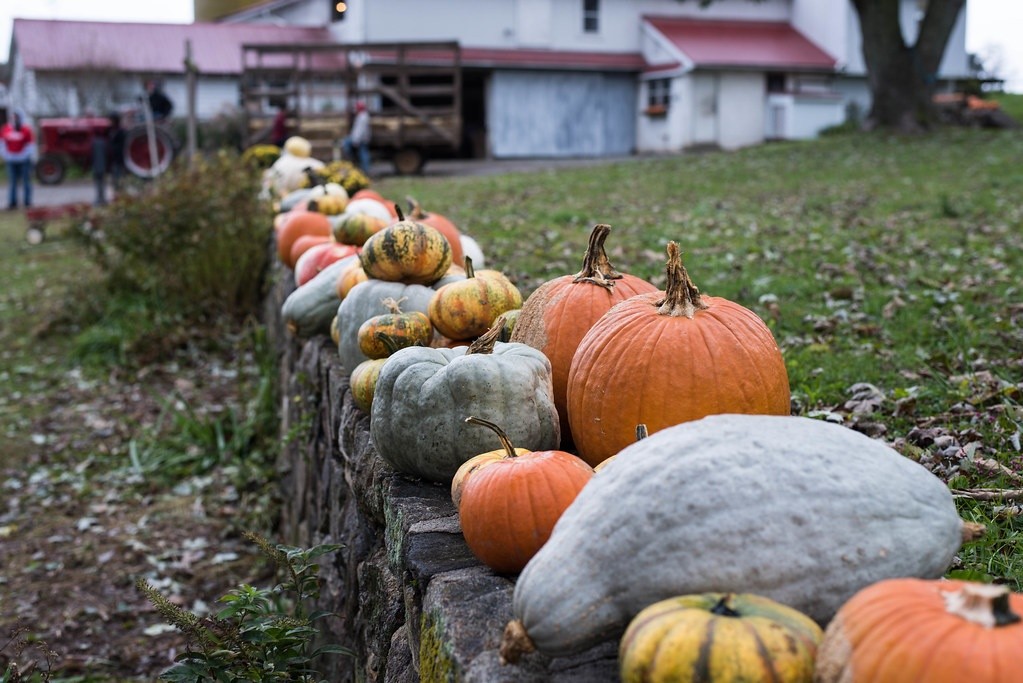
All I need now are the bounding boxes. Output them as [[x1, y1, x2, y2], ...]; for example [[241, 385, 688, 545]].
[[0, 112, 40, 212], [88, 110, 130, 206], [271, 102, 290, 148], [343, 100, 371, 169]]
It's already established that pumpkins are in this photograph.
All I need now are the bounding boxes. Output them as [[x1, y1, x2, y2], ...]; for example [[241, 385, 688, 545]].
[[264, 135, 1023, 683]]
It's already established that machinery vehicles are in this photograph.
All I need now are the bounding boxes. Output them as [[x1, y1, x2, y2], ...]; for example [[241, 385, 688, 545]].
[[34, 93, 182, 186]]
